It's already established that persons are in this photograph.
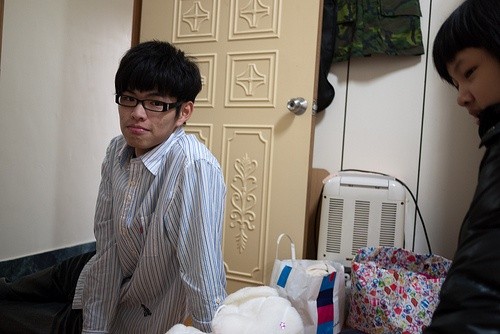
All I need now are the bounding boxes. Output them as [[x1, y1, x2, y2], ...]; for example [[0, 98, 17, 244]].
[[422, 0, 500, 334], [2, 37, 228, 334]]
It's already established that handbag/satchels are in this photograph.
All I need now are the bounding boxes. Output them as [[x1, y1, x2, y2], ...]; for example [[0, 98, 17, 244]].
[[345, 244, 453, 334], [270, 234, 345, 334]]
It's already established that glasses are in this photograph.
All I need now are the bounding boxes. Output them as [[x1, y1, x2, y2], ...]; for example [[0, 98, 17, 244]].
[[114, 93, 185, 112]]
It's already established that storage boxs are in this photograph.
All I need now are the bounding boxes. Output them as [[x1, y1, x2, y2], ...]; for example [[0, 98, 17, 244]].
[[267, 234, 346, 334], [345, 246, 453, 333]]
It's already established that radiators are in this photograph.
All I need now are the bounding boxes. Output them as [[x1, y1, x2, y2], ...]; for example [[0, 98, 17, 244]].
[[313, 170, 406, 271]]
